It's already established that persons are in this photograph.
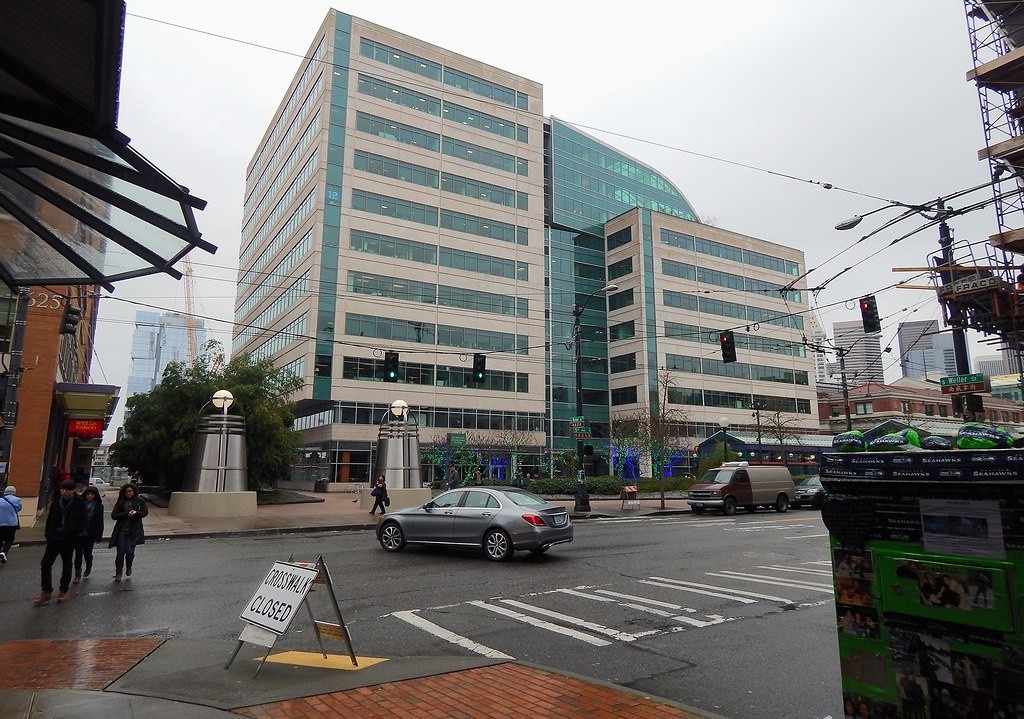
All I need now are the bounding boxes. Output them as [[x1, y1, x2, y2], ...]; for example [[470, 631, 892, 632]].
[[835, 552, 1024, 719], [516, 470, 523, 488], [472, 467, 481, 486], [0, 485, 22, 564], [32, 479, 87, 605], [52, 467, 89, 503], [524, 472, 533, 478], [688, 475, 691, 479], [73, 486, 104, 584], [127, 473, 138, 484], [109, 483, 149, 581], [369, 475, 386, 515], [445, 464, 458, 491]]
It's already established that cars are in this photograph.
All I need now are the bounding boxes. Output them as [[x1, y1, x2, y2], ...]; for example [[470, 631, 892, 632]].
[[789, 476, 826, 509], [376, 485, 574, 563]]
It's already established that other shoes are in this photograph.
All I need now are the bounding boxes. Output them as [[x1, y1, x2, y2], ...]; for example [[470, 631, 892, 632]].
[[73, 577, 82, 584], [84, 569, 91, 577], [57, 592, 68, 602], [369, 511, 375, 514], [379, 512, 385, 515], [115, 572, 122, 582], [32, 592, 52, 606], [126, 567, 132, 576], [0, 552, 7, 564]]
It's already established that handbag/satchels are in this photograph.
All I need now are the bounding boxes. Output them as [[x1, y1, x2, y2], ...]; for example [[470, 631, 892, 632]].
[[16, 515, 21, 530], [371, 491, 376, 496], [385, 498, 390, 506]]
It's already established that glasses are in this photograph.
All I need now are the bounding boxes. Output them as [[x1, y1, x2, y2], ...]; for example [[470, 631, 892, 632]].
[[125, 489, 133, 493]]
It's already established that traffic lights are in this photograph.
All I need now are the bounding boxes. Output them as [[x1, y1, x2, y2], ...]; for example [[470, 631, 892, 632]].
[[63, 305, 82, 335], [475, 355, 486, 381], [719, 335, 732, 359], [386, 354, 397, 381], [859, 300, 876, 330]]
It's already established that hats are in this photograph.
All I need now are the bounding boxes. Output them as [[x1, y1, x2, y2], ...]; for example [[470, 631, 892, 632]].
[[4, 486, 16, 495], [449, 464, 455, 467]]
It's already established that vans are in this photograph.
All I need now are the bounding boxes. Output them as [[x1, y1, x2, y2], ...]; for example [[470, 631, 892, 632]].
[[687, 460, 795, 516]]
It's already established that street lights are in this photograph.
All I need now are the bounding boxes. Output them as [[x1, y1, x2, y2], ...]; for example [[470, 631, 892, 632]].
[[573, 284, 619, 470], [834, 197, 971, 375]]
